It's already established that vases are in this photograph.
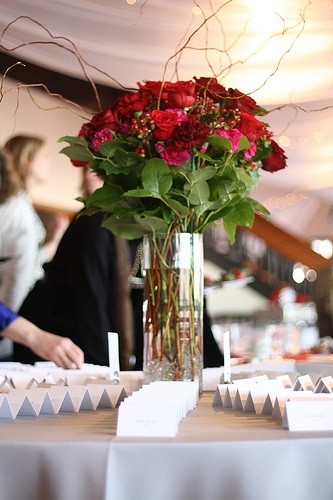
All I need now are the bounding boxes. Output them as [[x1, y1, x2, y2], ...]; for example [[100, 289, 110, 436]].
[[143, 233, 205, 395]]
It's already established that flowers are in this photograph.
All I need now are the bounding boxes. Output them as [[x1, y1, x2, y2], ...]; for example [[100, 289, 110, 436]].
[[57, 77, 288, 380]]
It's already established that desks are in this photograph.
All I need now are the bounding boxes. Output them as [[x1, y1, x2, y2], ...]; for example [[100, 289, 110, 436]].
[[0, 391, 333, 500]]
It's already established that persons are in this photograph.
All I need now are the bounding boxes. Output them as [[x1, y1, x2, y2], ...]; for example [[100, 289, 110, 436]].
[[10, 205, 224, 370], [1, 132, 69, 359], [0, 299, 86, 371]]
